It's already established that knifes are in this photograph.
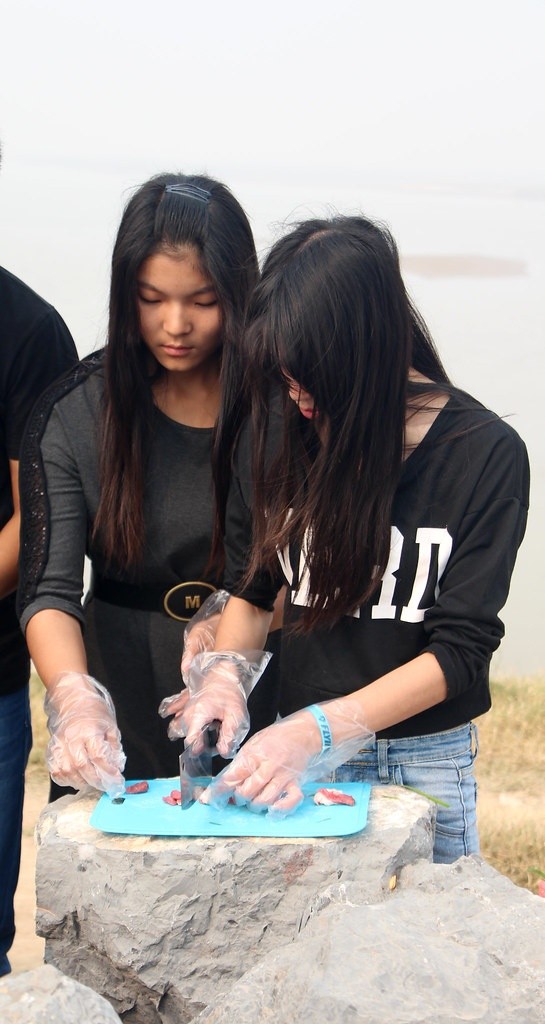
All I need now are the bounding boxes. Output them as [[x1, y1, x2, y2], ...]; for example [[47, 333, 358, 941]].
[[179, 719, 221, 810]]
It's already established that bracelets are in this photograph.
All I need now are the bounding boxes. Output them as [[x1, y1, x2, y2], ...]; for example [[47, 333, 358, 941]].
[[306, 705, 333, 764]]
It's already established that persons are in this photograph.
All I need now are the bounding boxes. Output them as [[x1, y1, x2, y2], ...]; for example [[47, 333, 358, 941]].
[[19, 171, 319, 803], [1, 267, 81, 981], [174, 212, 531, 861]]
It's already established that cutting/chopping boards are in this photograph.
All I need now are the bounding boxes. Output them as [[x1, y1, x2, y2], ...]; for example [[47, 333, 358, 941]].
[[89, 780, 372, 838]]
